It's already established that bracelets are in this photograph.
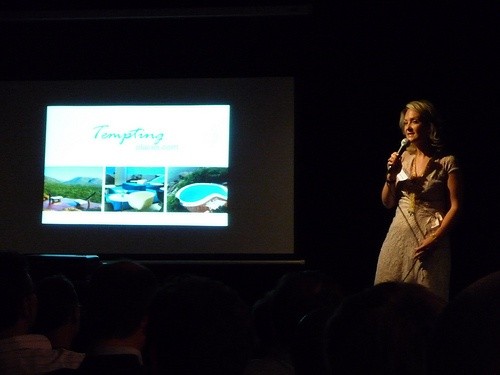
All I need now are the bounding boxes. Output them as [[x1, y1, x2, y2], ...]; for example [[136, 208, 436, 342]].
[[384, 177, 396, 184], [430, 233, 441, 241]]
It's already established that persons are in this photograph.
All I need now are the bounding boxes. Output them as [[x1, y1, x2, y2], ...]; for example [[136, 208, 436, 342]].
[[374, 98, 462, 302], [0, 249, 499, 374]]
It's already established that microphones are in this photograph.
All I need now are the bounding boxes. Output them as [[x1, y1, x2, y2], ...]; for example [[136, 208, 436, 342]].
[[388, 138, 410, 172]]
